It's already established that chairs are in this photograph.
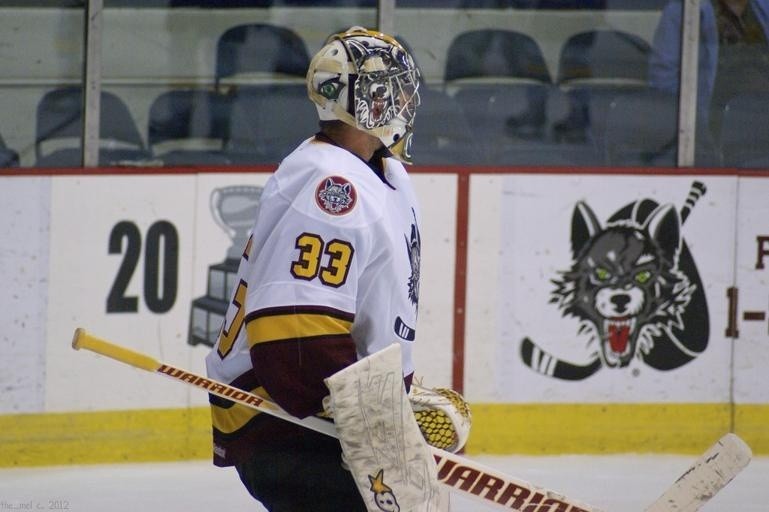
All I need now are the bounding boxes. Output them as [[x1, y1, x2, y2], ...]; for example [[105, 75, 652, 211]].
[[1, 25, 769, 166]]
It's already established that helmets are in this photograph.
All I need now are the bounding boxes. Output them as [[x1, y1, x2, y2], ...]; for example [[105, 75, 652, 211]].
[[306, 26, 422, 165]]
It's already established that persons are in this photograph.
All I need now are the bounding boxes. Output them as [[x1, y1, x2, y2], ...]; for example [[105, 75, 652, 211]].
[[203, 25, 476, 512], [645, 0, 722, 173]]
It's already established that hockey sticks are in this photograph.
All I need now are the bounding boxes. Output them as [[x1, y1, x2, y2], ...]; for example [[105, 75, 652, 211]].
[[72, 327, 753, 512]]
